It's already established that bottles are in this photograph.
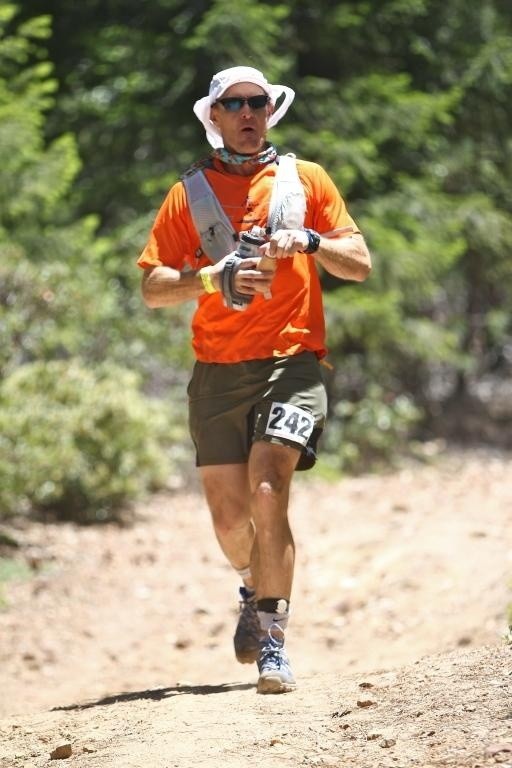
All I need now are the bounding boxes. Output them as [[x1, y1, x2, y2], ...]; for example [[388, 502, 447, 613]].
[[221, 226, 268, 311], [254, 248, 277, 293]]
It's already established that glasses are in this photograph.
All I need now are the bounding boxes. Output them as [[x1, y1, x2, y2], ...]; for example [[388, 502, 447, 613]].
[[211, 94, 270, 111]]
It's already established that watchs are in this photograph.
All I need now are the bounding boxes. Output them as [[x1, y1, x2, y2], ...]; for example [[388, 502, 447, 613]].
[[297, 228, 321, 255]]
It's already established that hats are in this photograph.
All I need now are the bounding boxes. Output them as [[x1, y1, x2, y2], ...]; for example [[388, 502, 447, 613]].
[[194, 67, 295, 151]]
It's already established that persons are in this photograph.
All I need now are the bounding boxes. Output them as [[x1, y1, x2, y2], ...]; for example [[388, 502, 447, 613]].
[[134, 63, 373, 695]]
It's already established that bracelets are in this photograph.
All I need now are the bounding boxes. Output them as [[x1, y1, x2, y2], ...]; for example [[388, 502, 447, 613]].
[[200, 265, 217, 294]]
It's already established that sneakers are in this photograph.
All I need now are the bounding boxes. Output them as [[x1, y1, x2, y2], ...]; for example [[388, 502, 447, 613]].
[[235, 584, 265, 663], [254, 640, 297, 692]]
